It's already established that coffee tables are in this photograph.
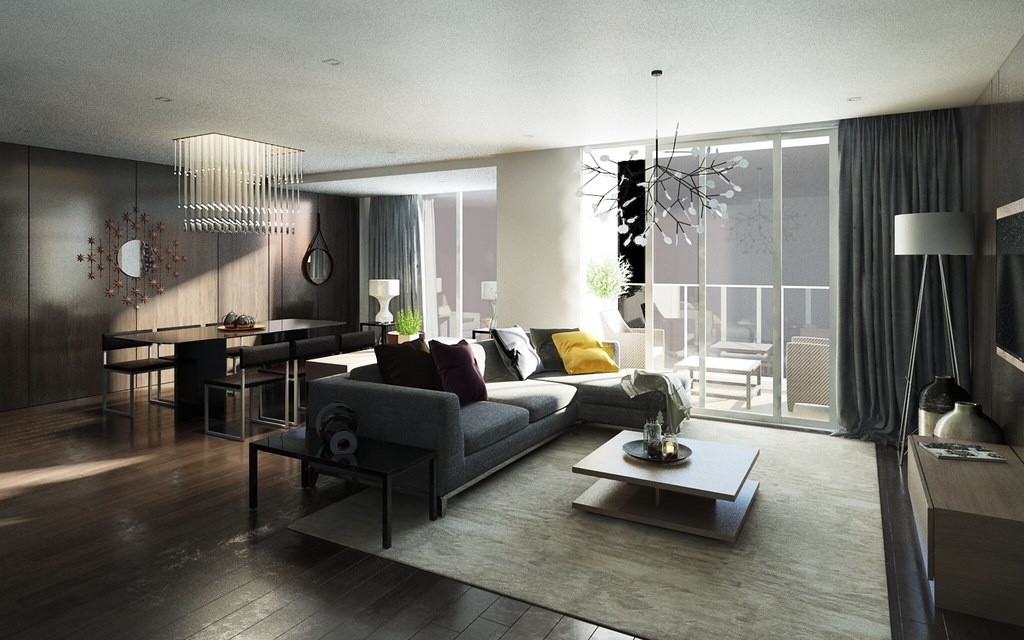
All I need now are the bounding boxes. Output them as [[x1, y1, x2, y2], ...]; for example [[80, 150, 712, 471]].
[[571, 430, 760, 544], [305, 348, 377, 382]]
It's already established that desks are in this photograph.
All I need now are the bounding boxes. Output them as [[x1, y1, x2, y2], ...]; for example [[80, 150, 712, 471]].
[[471, 328, 491, 340], [673, 354, 763, 410], [249, 426, 440, 549], [711, 342, 773, 376], [361, 321, 399, 344], [114, 318, 348, 437]]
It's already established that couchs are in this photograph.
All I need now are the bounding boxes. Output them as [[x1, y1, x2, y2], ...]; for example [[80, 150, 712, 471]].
[[301, 332, 691, 518]]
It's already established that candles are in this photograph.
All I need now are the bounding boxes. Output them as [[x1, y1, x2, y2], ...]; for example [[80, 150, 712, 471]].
[[666, 442, 674, 458]]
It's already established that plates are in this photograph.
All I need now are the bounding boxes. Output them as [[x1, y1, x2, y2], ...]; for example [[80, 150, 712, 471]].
[[218, 323, 265, 330], [623, 440, 692, 461]]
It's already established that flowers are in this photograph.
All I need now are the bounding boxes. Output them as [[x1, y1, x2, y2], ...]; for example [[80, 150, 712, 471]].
[[585, 251, 632, 303]]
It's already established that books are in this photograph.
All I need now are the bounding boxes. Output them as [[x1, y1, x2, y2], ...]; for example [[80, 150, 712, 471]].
[[917, 441, 1007, 463]]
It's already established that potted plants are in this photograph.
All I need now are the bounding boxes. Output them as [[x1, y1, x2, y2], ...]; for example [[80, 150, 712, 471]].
[[387, 307, 423, 345]]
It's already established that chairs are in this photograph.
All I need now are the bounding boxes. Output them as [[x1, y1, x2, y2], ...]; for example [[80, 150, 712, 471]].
[[599, 305, 666, 371], [100, 323, 375, 442], [786, 336, 831, 411], [642, 302, 697, 352]]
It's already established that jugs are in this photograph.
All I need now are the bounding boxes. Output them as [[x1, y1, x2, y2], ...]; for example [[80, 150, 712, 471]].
[[222, 310, 255, 328]]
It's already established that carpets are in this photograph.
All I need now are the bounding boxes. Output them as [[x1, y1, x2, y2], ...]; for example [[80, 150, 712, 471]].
[[289, 417, 893, 640]]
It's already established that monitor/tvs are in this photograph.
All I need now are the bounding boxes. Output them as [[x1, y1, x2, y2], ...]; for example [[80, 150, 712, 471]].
[[996, 197, 1024, 372]]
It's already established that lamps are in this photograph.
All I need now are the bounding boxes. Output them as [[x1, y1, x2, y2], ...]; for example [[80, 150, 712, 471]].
[[481, 281, 498, 316], [893, 213, 975, 464], [368, 279, 399, 324], [573, 70, 750, 248], [173, 132, 305, 237]]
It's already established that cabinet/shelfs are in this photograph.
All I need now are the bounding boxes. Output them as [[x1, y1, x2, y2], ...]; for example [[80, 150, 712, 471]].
[[907, 434, 1024, 630]]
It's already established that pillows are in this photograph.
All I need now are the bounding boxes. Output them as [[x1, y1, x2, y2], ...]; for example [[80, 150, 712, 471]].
[[372, 325, 619, 409]]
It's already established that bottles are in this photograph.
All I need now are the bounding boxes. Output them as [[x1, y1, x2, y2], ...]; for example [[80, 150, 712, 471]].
[[933, 401, 1006, 446], [918, 377, 971, 436], [662, 433, 679, 460], [644, 418, 661, 453]]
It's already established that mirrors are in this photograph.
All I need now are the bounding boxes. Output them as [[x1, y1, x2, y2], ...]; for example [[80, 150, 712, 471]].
[[301, 211, 335, 287]]
